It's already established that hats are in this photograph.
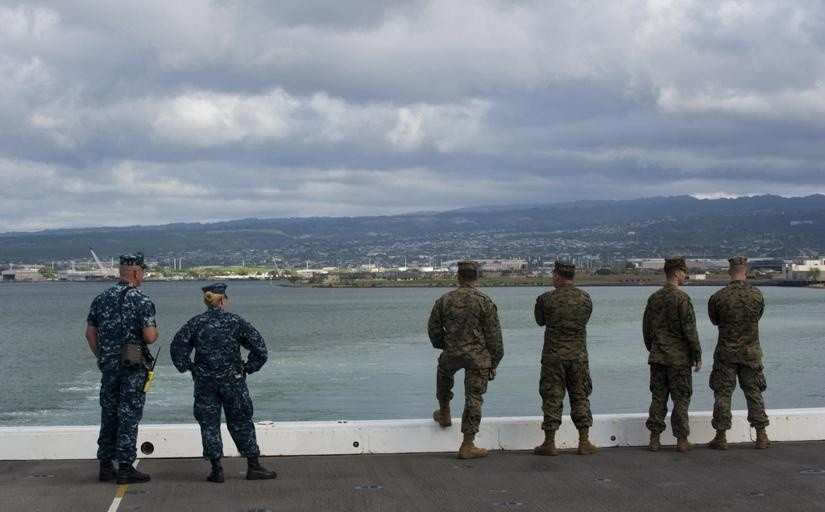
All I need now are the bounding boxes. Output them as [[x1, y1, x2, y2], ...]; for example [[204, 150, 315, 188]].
[[457, 260, 478, 270], [202, 283, 228, 299], [665, 257, 686, 271], [119, 252, 149, 270], [729, 257, 747, 265], [551, 261, 575, 273]]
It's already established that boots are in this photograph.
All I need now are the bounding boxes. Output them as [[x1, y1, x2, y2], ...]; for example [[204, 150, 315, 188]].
[[247, 459, 276, 479], [99, 462, 117, 481], [207, 460, 224, 482], [458, 441, 488, 458], [676, 439, 691, 451], [118, 464, 150, 484], [650, 433, 662, 450], [756, 431, 775, 449], [578, 441, 597, 454], [710, 430, 727, 450], [433, 409, 451, 425], [535, 437, 558, 455]]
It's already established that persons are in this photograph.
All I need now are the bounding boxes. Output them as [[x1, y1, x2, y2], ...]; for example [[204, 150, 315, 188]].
[[706, 255, 774, 450], [534, 259, 599, 456], [83, 250, 159, 483], [642, 254, 703, 452], [428, 261, 505, 460], [170, 284, 278, 481]]
